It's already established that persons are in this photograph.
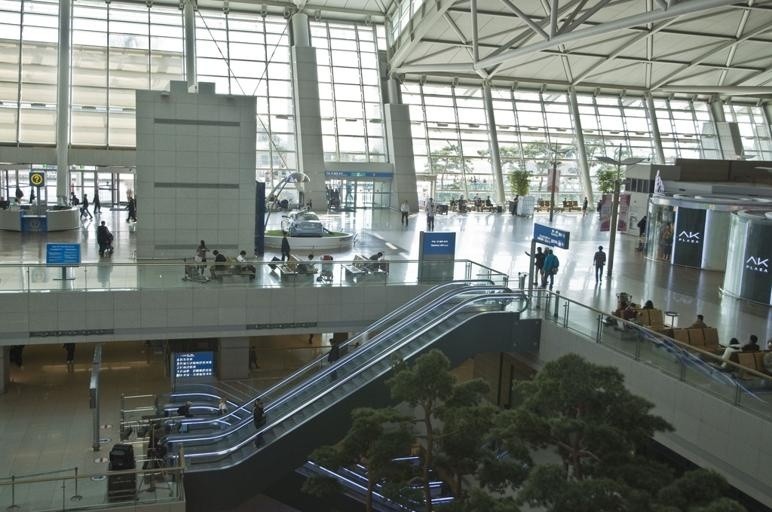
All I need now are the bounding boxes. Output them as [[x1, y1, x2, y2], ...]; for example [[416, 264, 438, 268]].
[[249, 345, 260, 369], [722, 335, 772, 376], [329, 339, 339, 381], [606, 300, 653, 327], [597, 200, 602, 211], [9, 345, 24, 369], [582, 197, 588, 216], [64, 344, 75, 373], [326, 182, 353, 210], [306, 199, 312, 212], [593, 246, 606, 282], [512, 195, 518, 215], [637, 206, 675, 259], [1, 185, 136, 256], [270, 194, 281, 206], [689, 314, 707, 328], [425, 198, 436, 231], [400, 200, 410, 226], [196, 232, 383, 271], [143, 397, 268, 484], [563, 198, 567, 207], [525, 247, 559, 290], [450, 193, 493, 213]]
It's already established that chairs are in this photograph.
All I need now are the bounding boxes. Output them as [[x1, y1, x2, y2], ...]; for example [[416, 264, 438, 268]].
[[448, 200, 496, 213], [342, 253, 387, 285], [275, 256, 317, 284], [534, 199, 581, 213], [636, 308, 772, 390], [213, 257, 255, 284]]
